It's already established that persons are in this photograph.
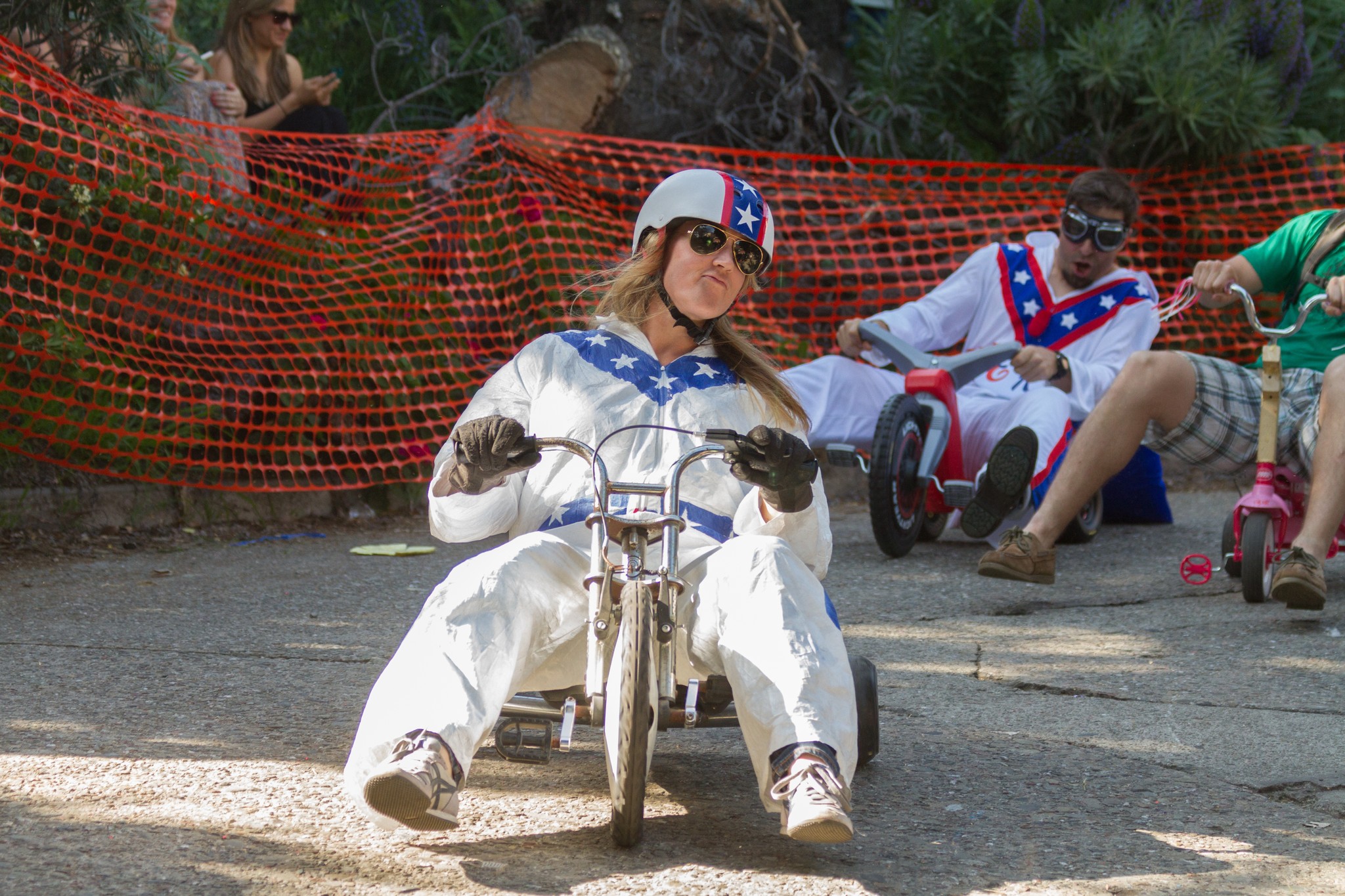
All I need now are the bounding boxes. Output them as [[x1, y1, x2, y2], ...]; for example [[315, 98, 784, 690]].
[[117, 1, 262, 227], [773, 169, 1174, 541], [346, 167, 858, 844], [203, 0, 355, 194], [0, 0, 121, 113], [977, 209, 1345, 608]]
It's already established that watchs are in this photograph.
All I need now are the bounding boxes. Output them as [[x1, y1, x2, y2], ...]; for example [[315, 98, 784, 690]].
[[1049, 350, 1070, 381]]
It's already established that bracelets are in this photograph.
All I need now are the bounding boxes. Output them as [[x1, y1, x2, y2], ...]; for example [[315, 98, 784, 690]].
[[277, 103, 289, 118]]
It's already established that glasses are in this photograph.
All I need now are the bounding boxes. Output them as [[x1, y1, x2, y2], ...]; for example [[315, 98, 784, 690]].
[[672, 223, 764, 276], [1060, 204, 1129, 252]]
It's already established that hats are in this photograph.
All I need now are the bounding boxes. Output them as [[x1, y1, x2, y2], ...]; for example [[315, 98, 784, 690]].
[[633, 169, 774, 345]]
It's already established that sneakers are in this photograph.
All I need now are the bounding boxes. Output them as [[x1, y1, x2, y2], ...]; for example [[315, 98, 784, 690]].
[[978, 526, 1055, 585], [364, 727, 459, 831], [960, 425, 1038, 538], [1271, 545, 1326, 610], [770, 761, 854, 844]]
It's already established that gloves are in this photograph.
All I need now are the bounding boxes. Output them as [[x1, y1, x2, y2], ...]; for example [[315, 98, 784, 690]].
[[447, 415, 542, 495], [730, 425, 819, 512]]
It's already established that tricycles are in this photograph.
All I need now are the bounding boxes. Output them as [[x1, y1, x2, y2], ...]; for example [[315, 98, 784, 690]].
[[836, 312, 1110, 563], [1173, 271, 1345, 608], [440, 412, 884, 856]]
